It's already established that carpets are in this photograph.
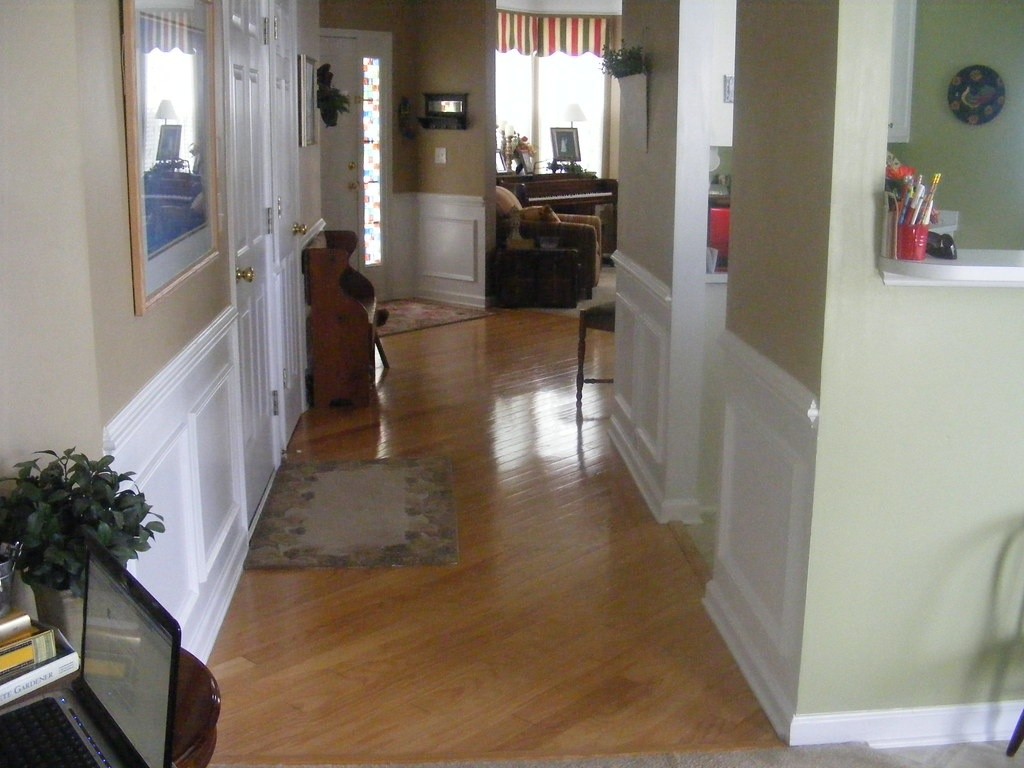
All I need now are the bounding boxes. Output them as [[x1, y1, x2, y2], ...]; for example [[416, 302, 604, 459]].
[[376, 297, 496, 337], [207, 740, 885, 768], [243, 453, 459, 569], [494, 263, 618, 318]]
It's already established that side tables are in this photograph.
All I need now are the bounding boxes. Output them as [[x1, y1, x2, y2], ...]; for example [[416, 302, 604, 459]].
[[496, 241, 592, 308]]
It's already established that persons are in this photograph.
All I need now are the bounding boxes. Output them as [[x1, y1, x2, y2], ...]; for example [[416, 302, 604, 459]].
[[560, 137, 567, 153]]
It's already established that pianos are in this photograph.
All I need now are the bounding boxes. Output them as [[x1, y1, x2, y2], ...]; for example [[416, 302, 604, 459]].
[[496, 171, 619, 216], [301, 229, 379, 411]]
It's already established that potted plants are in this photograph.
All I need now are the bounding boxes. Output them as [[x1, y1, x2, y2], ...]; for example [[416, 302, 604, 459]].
[[143, 159, 190, 181], [601, 38, 647, 154], [0, 447, 165, 658], [317, 64, 351, 128], [546, 160, 583, 173]]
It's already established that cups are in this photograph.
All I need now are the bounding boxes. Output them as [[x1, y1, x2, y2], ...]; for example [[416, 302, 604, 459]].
[[706, 247, 717, 274], [710, 207, 729, 274], [899, 223, 930, 261]]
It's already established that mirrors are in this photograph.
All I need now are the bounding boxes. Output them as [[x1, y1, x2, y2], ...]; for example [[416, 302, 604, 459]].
[[520, 204, 561, 222], [417, 92, 468, 129]]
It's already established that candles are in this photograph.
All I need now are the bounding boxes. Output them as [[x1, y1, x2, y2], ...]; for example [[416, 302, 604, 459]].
[[499, 121, 515, 136]]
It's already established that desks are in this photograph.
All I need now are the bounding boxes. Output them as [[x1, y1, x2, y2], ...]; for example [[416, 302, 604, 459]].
[[0, 646, 221, 768], [302, 230, 377, 410], [576, 307, 616, 400]]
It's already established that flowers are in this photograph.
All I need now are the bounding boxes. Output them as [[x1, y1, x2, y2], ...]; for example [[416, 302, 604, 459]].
[[189, 143, 203, 157], [512, 132, 537, 157]]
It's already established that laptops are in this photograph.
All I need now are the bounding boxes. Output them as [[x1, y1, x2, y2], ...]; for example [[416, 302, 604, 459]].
[[0, 526, 181, 768]]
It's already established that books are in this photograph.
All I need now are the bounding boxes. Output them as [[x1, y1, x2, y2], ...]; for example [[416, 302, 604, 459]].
[[0, 610, 79, 707]]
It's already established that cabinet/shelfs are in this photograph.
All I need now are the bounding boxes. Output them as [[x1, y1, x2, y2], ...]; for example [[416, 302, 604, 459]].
[[708, 0, 917, 147]]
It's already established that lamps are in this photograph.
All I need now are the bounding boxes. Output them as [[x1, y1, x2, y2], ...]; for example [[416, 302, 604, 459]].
[[564, 104, 587, 128], [154, 100, 177, 125]]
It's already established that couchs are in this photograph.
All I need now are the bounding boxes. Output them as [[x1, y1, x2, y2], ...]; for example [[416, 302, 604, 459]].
[[496, 185, 603, 300]]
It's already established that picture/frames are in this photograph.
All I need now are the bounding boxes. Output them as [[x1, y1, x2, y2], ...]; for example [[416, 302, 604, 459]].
[[550, 127, 581, 161], [122, 0, 220, 316], [495, 149, 507, 173], [156, 125, 182, 159], [297, 54, 318, 148]]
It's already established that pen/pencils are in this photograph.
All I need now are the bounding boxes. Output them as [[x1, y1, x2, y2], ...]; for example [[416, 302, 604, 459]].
[[897, 172, 941, 224]]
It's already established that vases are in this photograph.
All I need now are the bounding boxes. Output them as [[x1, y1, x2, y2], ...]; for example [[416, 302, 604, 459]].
[[193, 156, 202, 174], [518, 150, 533, 174]]
